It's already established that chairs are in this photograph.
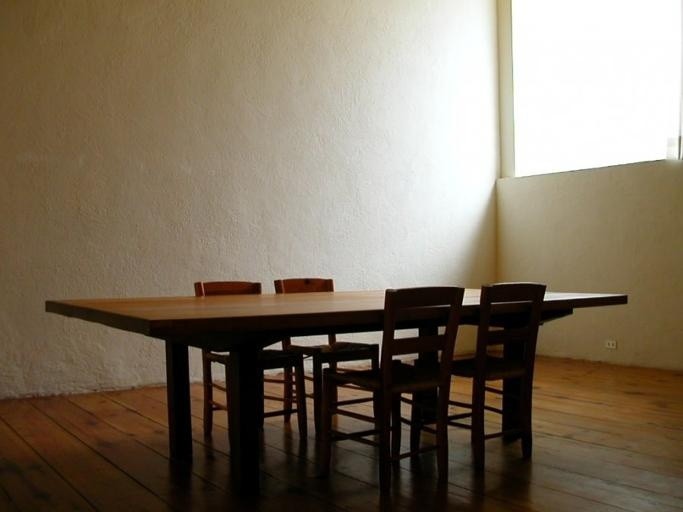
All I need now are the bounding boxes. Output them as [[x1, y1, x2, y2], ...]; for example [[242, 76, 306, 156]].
[[408, 281, 548, 465], [270, 275, 380, 459], [313, 288, 467, 500], [192, 278, 307, 445]]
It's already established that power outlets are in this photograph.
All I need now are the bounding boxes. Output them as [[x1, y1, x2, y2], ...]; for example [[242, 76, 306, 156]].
[[601, 337, 618, 349]]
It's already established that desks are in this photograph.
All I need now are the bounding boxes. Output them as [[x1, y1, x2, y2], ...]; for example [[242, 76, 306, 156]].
[[36, 287, 627, 506]]
